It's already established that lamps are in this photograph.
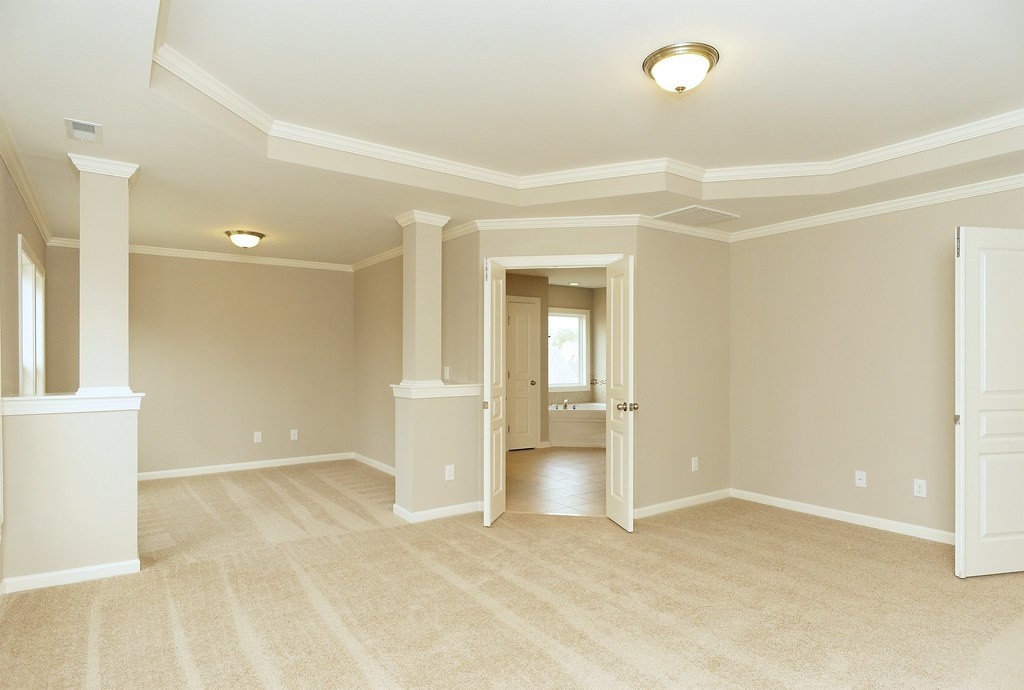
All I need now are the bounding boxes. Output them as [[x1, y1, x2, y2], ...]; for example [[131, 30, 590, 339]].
[[225, 230, 265, 248], [642, 41, 720, 94]]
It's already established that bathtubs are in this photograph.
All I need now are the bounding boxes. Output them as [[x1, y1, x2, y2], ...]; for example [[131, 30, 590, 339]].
[[548, 402, 606, 448]]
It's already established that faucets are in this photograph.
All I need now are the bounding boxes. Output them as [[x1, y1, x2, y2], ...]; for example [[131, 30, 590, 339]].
[[563, 399, 568, 410]]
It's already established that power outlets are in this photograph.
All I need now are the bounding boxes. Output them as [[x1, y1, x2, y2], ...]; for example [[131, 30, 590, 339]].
[[914, 479, 926, 497], [254, 432, 262, 443], [446, 464, 454, 480], [855, 471, 866, 488], [692, 457, 698, 472], [291, 429, 298, 440]]
